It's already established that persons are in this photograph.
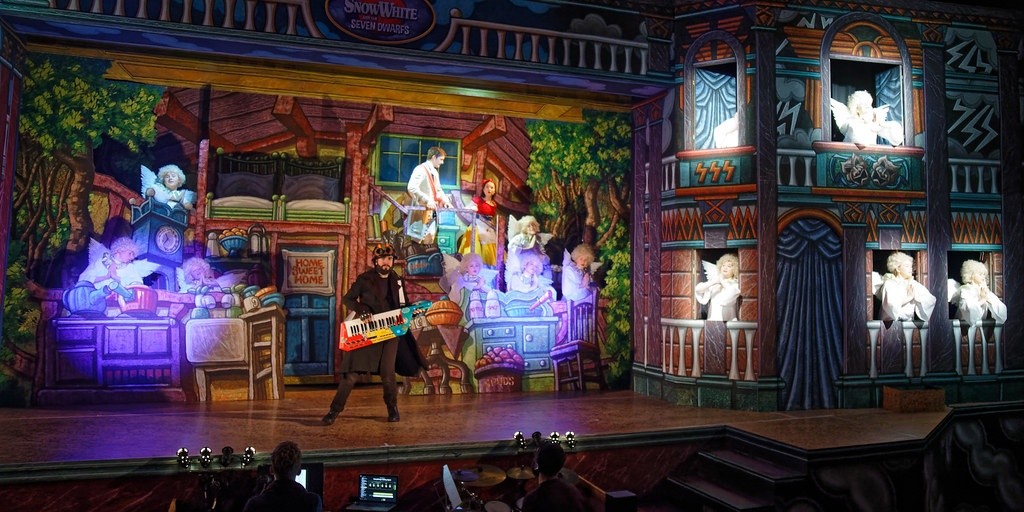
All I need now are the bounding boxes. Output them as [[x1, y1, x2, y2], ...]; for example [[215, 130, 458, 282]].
[[871, 251, 937, 322], [141, 164, 198, 210], [830, 90, 904, 147], [319, 242, 416, 426], [694, 253, 742, 322], [441, 253, 500, 304], [176, 256, 246, 294], [243, 440, 323, 512], [406, 146, 455, 209], [78, 236, 162, 290], [947, 259, 1007, 328], [521, 443, 585, 512], [458, 177, 507, 270], [504, 214, 557, 302], [562, 243, 605, 301], [713, 111, 739, 149]]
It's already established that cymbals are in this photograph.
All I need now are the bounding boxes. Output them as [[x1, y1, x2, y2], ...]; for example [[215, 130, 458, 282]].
[[445, 468, 478, 482], [555, 467, 579, 483], [507, 465, 536, 480], [462, 462, 507, 487]]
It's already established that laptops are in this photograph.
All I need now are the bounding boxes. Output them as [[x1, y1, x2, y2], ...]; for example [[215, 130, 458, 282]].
[[346, 474, 398, 512], [295, 468, 306, 492]]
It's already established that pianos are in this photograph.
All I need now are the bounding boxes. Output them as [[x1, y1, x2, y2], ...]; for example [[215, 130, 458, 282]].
[[338, 300, 432, 353]]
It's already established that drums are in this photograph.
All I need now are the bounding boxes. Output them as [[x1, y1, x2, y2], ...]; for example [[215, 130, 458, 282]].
[[482, 500, 512, 512], [514, 495, 525, 512]]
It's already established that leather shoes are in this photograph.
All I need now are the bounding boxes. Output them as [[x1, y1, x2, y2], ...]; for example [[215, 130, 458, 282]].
[[388, 406, 401, 422], [322, 411, 340, 425]]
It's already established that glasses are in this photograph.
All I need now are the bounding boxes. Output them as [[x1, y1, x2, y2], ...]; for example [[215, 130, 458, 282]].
[[374, 247, 391, 256]]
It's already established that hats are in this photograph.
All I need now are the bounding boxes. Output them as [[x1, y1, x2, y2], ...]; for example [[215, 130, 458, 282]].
[[372, 243, 398, 262]]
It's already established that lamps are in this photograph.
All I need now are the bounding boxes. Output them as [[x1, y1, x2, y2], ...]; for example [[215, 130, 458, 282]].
[[550, 431, 560, 444], [565, 430, 577, 448], [174, 444, 256, 467], [512, 430, 527, 448], [532, 432, 542, 447]]
